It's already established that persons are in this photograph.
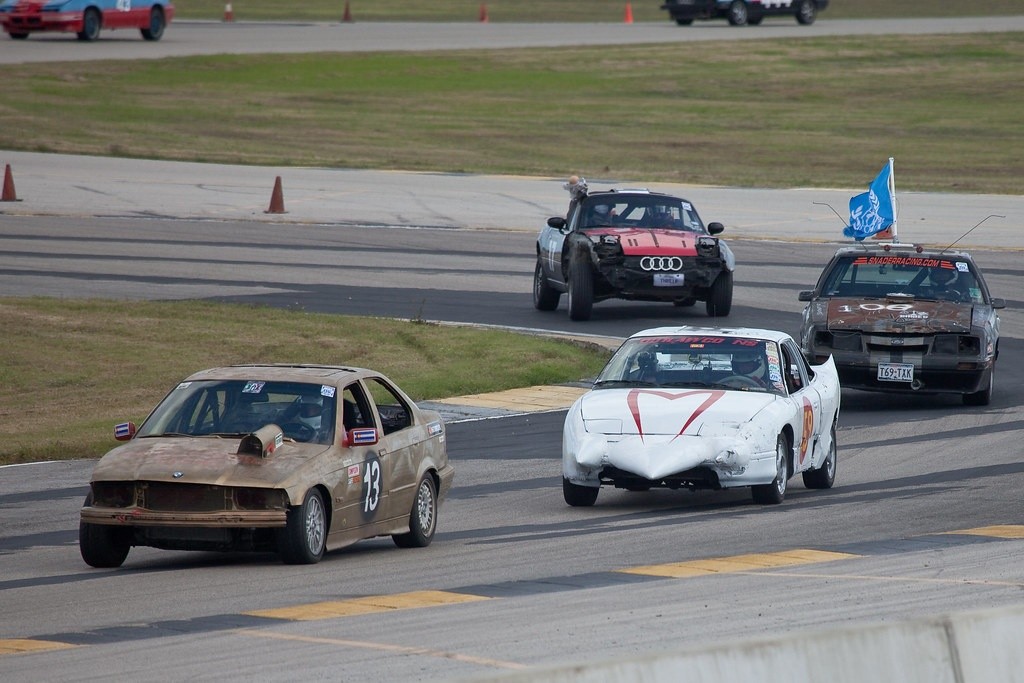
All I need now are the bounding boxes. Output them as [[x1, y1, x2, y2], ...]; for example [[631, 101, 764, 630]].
[[639, 200, 678, 228], [921, 255, 975, 301], [587, 196, 616, 227], [726, 350, 769, 391], [285, 393, 336, 444]]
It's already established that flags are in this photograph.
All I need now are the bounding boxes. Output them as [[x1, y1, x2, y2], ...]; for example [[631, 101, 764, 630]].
[[841, 156, 899, 243]]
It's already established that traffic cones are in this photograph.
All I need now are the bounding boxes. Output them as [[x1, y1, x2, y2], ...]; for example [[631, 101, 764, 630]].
[[264, 174, 290, 214], [222, 0, 235, 23], [623, 1, 635, 24], [477, 2, 489, 24], [340, 0, 356, 23], [0, 163, 21, 203]]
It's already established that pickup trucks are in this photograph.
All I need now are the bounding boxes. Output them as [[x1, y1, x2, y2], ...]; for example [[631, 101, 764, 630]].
[[533, 187, 736, 322]]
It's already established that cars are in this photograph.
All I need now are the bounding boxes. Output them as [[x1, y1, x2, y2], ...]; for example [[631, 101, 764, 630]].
[[798, 242, 1007, 407], [562, 325, 841, 507], [661, 0, 828, 28], [78, 362, 456, 568], [0, 0, 174, 42]]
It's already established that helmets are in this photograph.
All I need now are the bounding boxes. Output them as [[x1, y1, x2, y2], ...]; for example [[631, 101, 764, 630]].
[[730, 351, 766, 381], [929, 263, 959, 288], [647, 202, 672, 224], [296, 395, 323, 430]]
